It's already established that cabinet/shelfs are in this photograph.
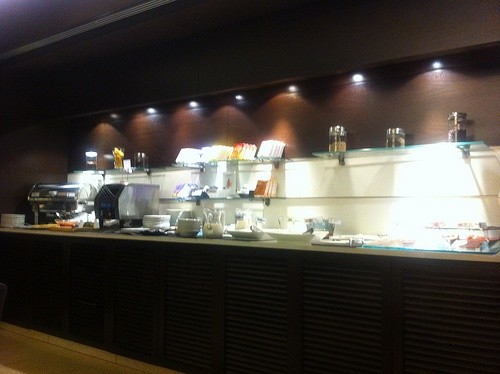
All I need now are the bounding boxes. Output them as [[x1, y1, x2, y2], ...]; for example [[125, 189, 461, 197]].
[[66, 142, 500, 247]]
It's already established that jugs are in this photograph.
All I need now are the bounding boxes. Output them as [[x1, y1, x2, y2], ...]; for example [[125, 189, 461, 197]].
[[203, 208, 223, 238]]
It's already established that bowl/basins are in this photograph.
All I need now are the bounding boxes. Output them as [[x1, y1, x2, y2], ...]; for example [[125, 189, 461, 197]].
[[176, 218, 201, 237]]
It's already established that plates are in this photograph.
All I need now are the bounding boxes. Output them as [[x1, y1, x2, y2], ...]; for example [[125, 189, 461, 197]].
[[228, 231, 262, 238], [142, 215, 170, 228], [1, 214, 25, 227]]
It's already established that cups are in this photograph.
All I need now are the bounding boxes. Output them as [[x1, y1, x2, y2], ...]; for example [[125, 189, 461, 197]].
[[329, 126, 347, 152], [385, 128, 404, 146]]
